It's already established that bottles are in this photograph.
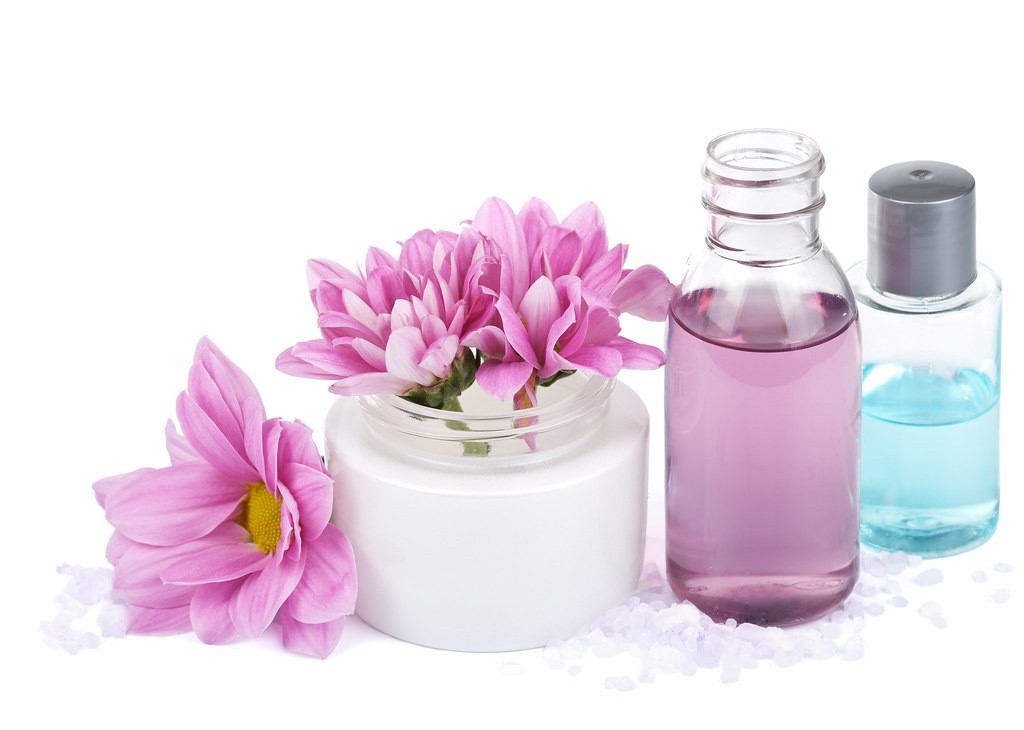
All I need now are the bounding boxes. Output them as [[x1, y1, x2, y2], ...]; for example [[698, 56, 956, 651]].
[[855, 161, 1002, 556], [665, 128, 864, 629]]
